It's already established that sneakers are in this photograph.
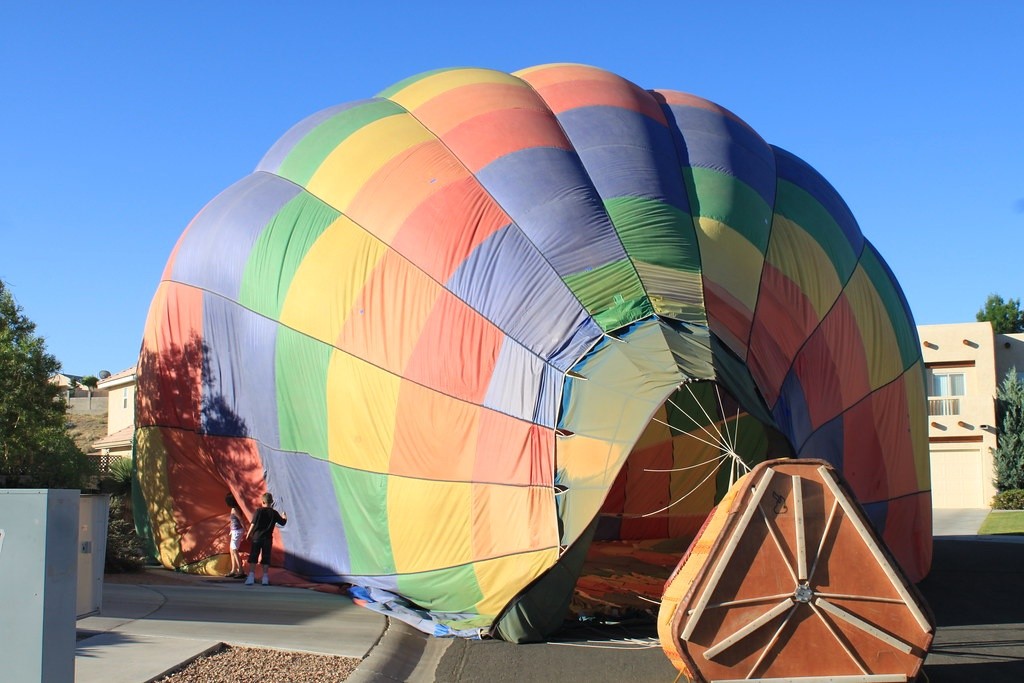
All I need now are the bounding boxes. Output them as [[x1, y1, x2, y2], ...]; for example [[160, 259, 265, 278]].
[[244, 573, 254, 584], [262, 577, 268, 585]]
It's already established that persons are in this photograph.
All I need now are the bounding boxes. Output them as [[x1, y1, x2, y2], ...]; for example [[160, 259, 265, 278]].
[[224, 492, 246, 579], [244, 493, 287, 585]]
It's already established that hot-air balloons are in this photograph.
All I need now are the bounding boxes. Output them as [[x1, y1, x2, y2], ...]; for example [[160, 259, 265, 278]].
[[128, 63, 936, 683]]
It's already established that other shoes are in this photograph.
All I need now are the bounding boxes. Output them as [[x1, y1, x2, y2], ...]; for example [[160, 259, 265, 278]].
[[233, 573, 245, 579], [225, 572, 235, 576]]
